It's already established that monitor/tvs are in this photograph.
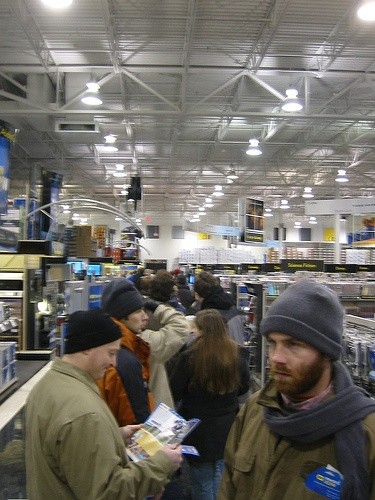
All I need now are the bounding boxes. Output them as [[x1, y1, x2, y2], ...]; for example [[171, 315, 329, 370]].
[[67, 261, 82, 274], [86, 264, 103, 276], [188, 273, 195, 284]]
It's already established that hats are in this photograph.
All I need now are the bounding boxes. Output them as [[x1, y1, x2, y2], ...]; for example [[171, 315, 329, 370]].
[[179, 289, 194, 307], [64, 309, 122, 354], [260, 278, 343, 361], [102, 279, 143, 320]]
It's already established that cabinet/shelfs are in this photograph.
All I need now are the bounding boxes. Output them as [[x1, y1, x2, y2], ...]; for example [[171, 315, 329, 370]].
[[205, 271, 375, 399], [0, 250, 138, 355]]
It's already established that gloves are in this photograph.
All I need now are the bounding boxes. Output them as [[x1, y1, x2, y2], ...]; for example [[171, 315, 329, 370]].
[[143, 298, 158, 313]]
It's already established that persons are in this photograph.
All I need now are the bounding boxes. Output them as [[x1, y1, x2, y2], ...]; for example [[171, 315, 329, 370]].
[[170, 309, 248, 500], [193, 271, 245, 347], [24, 308, 185, 500], [137, 295, 190, 415], [122, 266, 238, 312], [96, 279, 156, 427], [218, 278, 374, 499], [149, 270, 193, 412]]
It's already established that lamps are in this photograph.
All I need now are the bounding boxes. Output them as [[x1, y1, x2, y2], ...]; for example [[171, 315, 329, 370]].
[[81, 80, 105, 107], [103, 134, 120, 155], [247, 136, 264, 155], [282, 72, 304, 114], [188, 165, 351, 230]]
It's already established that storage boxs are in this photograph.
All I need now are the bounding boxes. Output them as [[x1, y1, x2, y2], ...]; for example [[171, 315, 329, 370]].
[[178, 241, 375, 265], [65, 225, 98, 258]]
